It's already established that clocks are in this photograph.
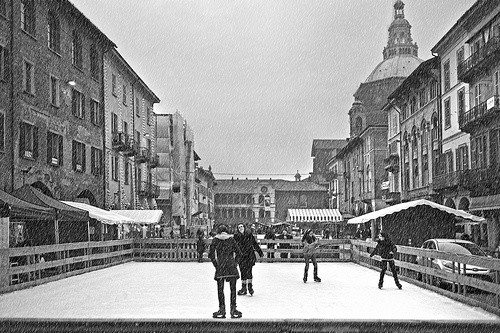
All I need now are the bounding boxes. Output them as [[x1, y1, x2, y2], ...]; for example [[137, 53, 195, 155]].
[[261, 186, 267, 193]]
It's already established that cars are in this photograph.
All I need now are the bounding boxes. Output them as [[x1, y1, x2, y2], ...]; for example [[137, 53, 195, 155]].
[[415, 238, 495, 294]]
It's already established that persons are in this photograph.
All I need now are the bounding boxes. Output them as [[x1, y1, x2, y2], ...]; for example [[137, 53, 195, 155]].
[[301, 229, 321, 283], [250, 224, 372, 258], [234, 223, 264, 297], [370, 233, 402, 289], [208, 224, 242, 318], [156, 227, 216, 263], [460, 230, 488, 247]]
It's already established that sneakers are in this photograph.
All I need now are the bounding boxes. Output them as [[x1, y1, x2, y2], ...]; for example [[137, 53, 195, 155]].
[[237, 283, 247, 295], [395, 280, 402, 290], [377, 280, 384, 289], [314, 274, 320, 282], [229, 303, 242, 319], [212, 305, 226, 319], [247, 283, 254, 296], [303, 273, 308, 283]]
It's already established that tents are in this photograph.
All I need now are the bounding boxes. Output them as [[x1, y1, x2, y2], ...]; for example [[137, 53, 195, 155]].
[[0, 182, 164, 274], [285, 208, 344, 239]]
